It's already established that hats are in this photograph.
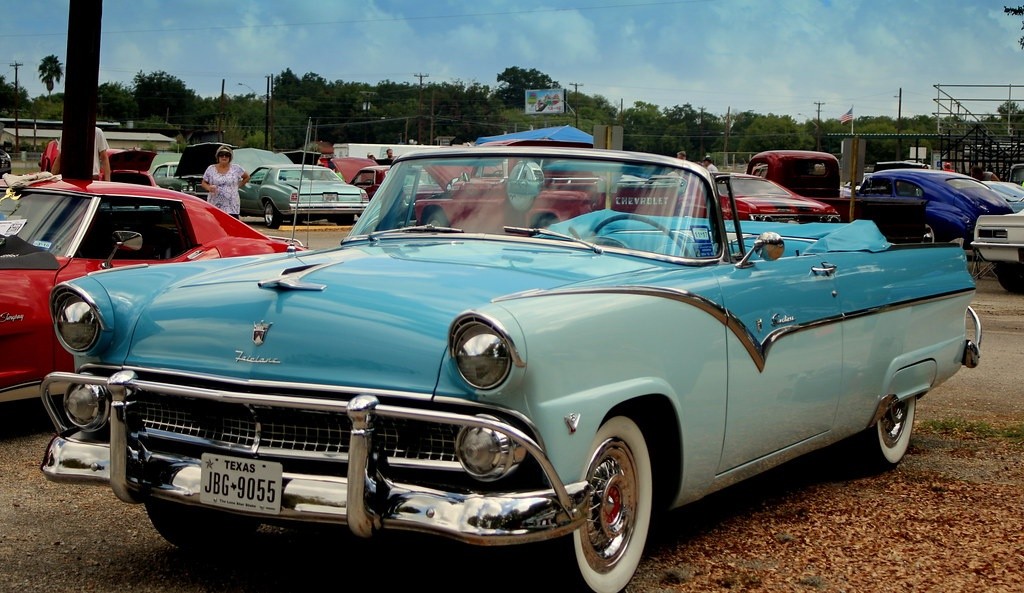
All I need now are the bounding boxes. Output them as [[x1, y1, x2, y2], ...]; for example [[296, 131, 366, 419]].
[[367, 152, 373, 157]]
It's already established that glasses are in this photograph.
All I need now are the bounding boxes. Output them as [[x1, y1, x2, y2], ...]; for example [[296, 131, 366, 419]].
[[218, 151, 230, 157]]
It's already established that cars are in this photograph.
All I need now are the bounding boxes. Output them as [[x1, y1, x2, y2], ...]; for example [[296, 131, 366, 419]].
[[0, 177, 310, 402], [981, 181, 1024, 212], [330, 158, 445, 220], [230, 148, 369, 229], [151, 142, 239, 201], [98, 149, 156, 186], [852, 168, 1013, 242], [971, 209, 1024, 291], [678, 171, 841, 223], [414, 159, 686, 239]]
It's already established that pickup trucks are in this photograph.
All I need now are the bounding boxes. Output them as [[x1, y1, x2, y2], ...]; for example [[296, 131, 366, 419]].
[[745, 150, 927, 243], [37, 147, 982, 593]]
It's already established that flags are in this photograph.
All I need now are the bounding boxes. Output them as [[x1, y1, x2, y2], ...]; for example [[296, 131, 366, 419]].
[[841, 108, 853, 124]]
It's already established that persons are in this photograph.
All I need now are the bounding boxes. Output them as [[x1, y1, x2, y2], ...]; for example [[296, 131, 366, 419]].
[[202, 146, 250, 220], [676, 151, 718, 177], [942, 161, 954, 171], [970, 166, 1001, 182], [52, 126, 110, 182], [366, 148, 396, 166]]
